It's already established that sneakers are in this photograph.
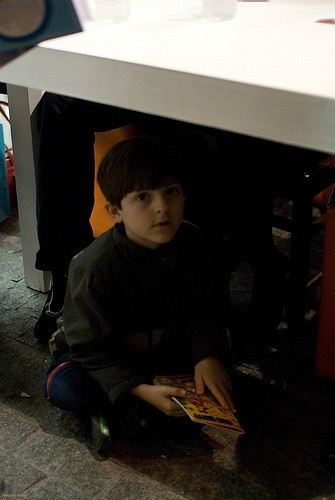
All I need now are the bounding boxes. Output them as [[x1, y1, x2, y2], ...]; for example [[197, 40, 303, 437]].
[[91, 413, 113, 454]]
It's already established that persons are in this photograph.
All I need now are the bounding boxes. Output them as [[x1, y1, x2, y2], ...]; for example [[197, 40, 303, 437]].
[[39, 139, 291, 454], [32, 94, 142, 339], [1, 1, 147, 344]]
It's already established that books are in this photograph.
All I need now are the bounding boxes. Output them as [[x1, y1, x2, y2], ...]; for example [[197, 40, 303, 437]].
[[154, 373, 245, 438]]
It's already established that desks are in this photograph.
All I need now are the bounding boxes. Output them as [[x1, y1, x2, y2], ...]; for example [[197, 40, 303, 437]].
[[0, 1, 335, 294]]
